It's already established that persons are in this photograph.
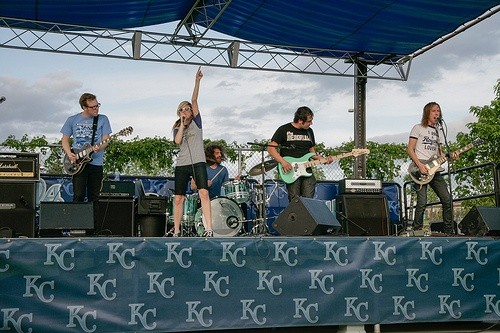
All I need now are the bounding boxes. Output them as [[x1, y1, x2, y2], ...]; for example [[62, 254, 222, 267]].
[[60, 93, 113, 237], [174, 66, 241, 236], [266, 106, 334, 203], [408, 101, 460, 233]]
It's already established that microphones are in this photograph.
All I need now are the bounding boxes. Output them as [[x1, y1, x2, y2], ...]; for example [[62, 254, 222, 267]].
[[436, 116, 443, 121], [182, 116, 185, 123], [20, 196, 28, 206]]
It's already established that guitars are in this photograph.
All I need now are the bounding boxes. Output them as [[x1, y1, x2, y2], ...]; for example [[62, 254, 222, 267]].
[[63, 126, 134, 176], [408, 137, 485, 185], [278, 147, 370, 183]]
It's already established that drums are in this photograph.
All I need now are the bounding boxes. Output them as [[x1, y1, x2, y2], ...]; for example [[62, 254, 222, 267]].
[[167, 194, 189, 224], [222, 179, 249, 204], [193, 196, 244, 237], [190, 193, 202, 225]]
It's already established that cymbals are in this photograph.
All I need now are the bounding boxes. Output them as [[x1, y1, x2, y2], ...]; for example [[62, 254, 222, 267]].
[[248, 159, 278, 176]]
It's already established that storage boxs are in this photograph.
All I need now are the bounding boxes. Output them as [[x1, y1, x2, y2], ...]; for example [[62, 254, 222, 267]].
[[138, 196, 168, 214]]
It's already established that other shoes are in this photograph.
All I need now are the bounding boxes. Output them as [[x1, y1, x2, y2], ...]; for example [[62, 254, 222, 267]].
[[173, 230, 181, 237], [202, 230, 213, 237], [445, 231, 459, 236]]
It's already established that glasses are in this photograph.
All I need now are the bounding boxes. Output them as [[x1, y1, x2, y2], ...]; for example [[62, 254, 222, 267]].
[[179, 107, 192, 111], [87, 103, 101, 110]]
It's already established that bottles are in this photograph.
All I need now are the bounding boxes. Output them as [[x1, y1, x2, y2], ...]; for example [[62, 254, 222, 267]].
[[115, 168, 120, 181]]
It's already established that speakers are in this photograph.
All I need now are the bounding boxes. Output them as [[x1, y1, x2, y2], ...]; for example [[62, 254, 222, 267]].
[[96, 199, 134, 237], [344, 195, 388, 236], [272, 196, 342, 237], [458, 205, 500, 236], [0, 183, 35, 237], [39, 201, 95, 236]]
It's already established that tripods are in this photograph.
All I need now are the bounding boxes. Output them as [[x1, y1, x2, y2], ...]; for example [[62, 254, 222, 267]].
[[247, 142, 290, 235]]
